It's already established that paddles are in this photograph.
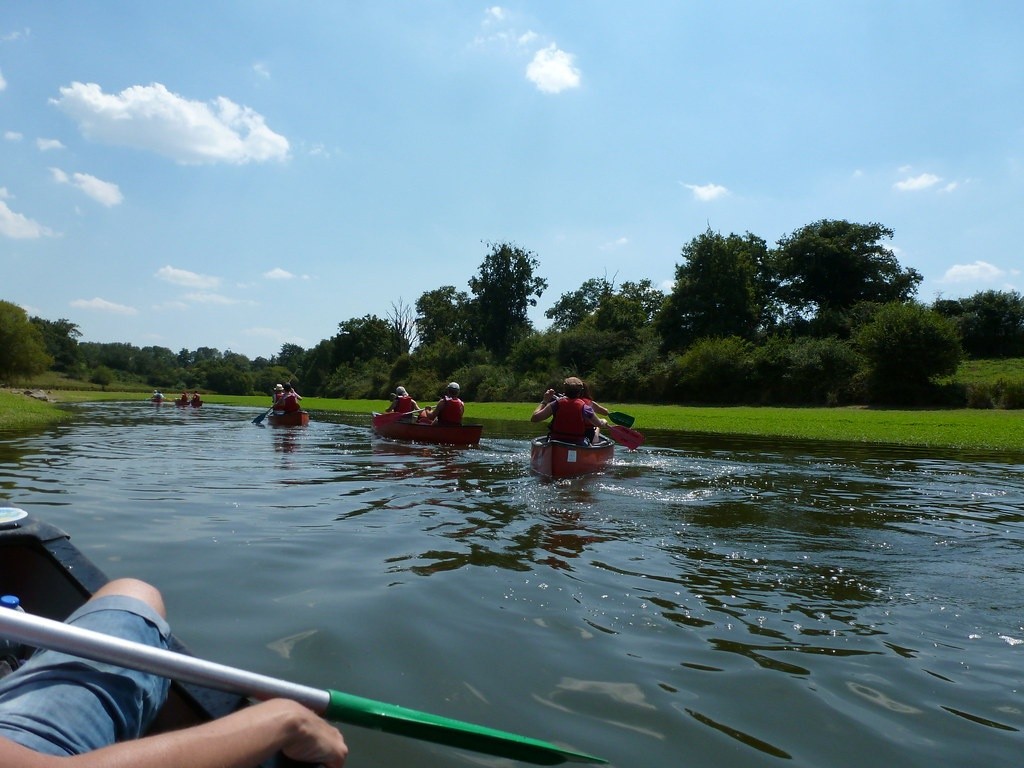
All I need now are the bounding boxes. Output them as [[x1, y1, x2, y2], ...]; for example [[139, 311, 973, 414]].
[[250, 394, 285, 424], [552, 393, 645, 451], [372, 405, 438, 429], [1, 604, 612, 768], [557, 391, 636, 429]]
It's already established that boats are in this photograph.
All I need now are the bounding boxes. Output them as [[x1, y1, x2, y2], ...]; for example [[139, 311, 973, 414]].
[[190, 400, 204, 408], [268, 410, 309, 426], [151, 398, 163, 403], [531, 433, 615, 478], [372, 411, 483, 446], [175, 398, 190, 406]]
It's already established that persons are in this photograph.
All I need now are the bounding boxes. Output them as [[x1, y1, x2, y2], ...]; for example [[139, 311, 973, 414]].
[[531, 377, 607, 445], [272, 382, 302, 415], [425, 382, 464, 426], [193, 390, 200, 401], [0, 579, 348, 768], [385, 386, 422, 420], [182, 393, 189, 401], [153, 390, 163, 398]]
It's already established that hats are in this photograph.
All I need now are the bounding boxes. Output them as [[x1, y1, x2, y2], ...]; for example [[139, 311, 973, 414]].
[[564, 377, 584, 390], [274, 384, 284, 390], [283, 382, 291, 389], [396, 386, 409, 397], [447, 382, 460, 389]]
